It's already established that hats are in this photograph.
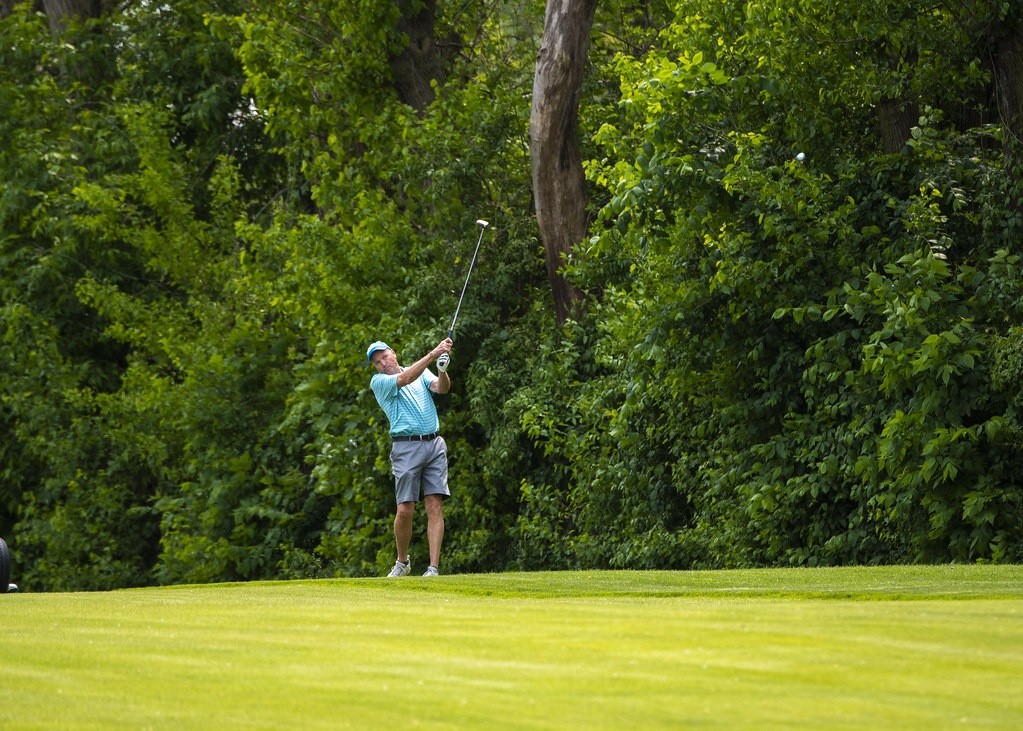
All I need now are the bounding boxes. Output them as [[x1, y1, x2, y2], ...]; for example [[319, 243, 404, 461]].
[[367, 340, 392, 362]]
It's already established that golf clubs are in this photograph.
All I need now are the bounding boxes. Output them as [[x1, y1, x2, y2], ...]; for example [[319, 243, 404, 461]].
[[437, 219, 491, 367]]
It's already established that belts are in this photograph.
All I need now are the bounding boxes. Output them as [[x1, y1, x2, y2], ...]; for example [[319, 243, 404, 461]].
[[393, 431, 439, 442]]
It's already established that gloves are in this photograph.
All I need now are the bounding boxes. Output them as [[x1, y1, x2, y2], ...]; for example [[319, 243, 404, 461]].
[[436, 352, 450, 372]]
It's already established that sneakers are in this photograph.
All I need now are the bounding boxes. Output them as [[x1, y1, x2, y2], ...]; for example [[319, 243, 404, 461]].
[[423, 565, 438, 576], [387, 554, 411, 577]]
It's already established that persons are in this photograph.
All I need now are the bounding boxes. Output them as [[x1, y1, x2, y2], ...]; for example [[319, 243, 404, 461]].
[[366, 338, 453, 577]]
